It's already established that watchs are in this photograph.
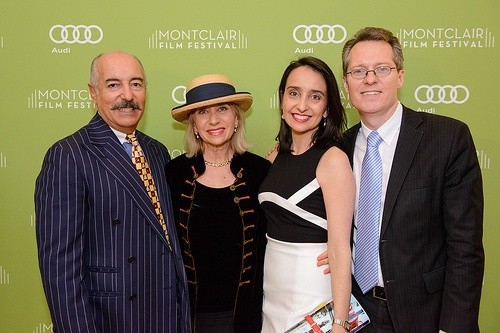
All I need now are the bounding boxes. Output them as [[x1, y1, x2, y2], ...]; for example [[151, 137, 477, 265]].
[[333, 319, 350, 331]]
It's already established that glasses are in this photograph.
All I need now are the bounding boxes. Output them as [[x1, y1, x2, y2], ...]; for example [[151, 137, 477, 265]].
[[345, 65, 397, 80]]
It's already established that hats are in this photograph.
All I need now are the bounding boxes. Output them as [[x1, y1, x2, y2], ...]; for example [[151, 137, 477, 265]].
[[171, 74, 254, 124]]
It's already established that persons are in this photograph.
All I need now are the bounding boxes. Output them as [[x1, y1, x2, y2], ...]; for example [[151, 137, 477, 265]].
[[34, 52, 195, 333], [164, 73, 334, 333], [258, 56, 356, 332], [268, 27, 485, 333]]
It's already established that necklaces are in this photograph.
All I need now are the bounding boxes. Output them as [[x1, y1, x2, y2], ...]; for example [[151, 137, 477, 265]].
[[205, 155, 232, 168]]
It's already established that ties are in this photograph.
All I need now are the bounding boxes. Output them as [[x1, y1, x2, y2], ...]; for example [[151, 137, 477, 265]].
[[126, 133, 171, 248], [353, 132, 382, 294]]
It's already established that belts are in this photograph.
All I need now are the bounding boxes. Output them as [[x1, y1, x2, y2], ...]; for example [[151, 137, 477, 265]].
[[354, 279, 388, 301]]
